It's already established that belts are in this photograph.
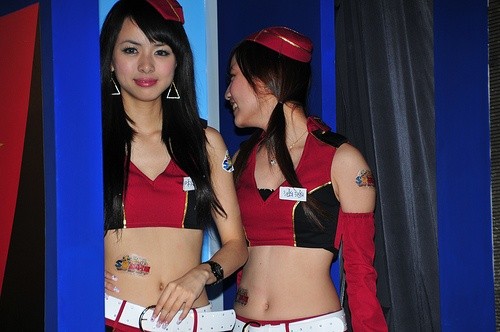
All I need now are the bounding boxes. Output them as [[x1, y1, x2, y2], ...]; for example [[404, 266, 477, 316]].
[[231, 307, 348, 332], [104, 294, 236, 332]]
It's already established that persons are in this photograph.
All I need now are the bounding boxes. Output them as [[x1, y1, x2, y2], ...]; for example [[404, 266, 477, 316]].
[[225, 26, 388, 332], [100, 0, 247, 330]]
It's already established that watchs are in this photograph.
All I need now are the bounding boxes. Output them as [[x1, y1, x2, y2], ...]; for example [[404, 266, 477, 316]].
[[203, 258, 224, 286]]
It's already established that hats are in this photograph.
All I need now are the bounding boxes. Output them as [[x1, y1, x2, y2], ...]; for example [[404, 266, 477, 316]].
[[245, 25, 314, 63], [145, 0, 185, 24]]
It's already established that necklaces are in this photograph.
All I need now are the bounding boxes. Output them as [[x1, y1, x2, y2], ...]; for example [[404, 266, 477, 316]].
[[269, 128, 308, 164]]
